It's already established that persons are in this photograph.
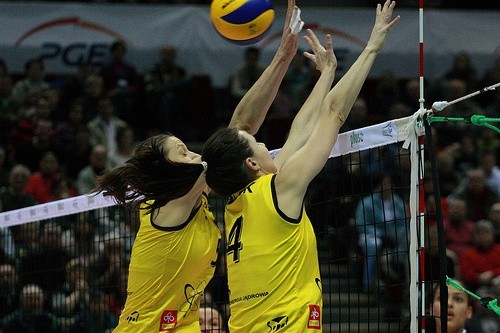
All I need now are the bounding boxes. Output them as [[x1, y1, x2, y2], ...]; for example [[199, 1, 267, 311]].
[[199, 302, 227, 333], [344, 97, 368, 133], [143, 45, 186, 97], [0, 59, 134, 333], [231, 46, 264, 96], [440, 51, 478, 79], [354, 79, 500, 333], [98, 42, 136, 95], [92, 0, 306, 333], [132, 0, 402, 333]]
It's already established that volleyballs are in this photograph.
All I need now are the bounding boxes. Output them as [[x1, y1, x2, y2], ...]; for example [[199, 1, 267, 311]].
[[210, 0, 276, 44]]
